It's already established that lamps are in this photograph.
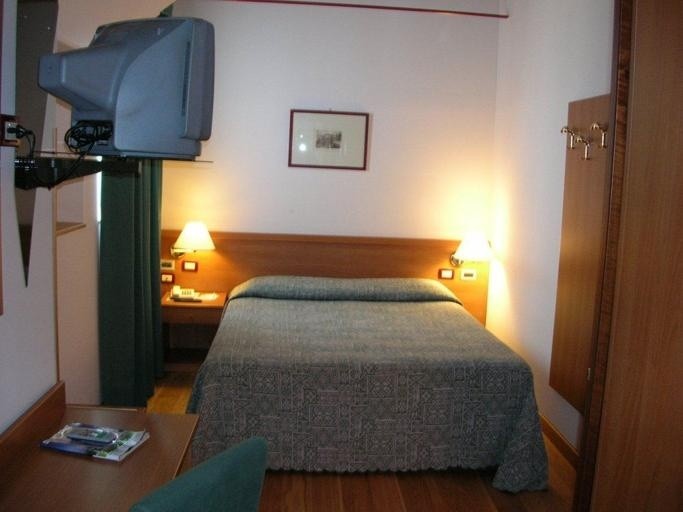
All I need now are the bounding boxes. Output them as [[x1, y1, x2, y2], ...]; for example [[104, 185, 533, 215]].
[[170, 222, 216, 259], [450, 232, 495, 268]]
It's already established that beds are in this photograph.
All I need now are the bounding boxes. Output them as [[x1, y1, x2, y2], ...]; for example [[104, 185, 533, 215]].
[[182, 296, 548, 495]]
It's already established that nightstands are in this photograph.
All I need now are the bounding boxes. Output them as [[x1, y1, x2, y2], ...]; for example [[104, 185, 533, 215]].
[[160, 290, 228, 373]]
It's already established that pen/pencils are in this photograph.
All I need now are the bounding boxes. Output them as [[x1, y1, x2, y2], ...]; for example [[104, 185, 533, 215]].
[[174, 299, 200, 301]]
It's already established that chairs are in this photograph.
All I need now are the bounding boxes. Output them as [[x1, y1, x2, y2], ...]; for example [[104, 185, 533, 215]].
[[127, 436, 269, 512]]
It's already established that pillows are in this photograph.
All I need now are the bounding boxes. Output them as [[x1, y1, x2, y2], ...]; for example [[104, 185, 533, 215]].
[[230, 275, 464, 307]]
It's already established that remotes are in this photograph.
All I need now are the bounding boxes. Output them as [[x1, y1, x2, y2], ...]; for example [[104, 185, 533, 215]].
[[67, 427, 117, 444]]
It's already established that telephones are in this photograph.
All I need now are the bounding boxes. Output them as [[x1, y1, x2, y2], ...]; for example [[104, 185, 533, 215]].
[[170, 285, 194, 299]]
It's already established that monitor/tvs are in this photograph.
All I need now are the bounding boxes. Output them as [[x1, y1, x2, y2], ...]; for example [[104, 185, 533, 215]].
[[38, 15, 215, 160]]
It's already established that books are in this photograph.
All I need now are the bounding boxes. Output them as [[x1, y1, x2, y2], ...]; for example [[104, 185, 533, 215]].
[[41, 423, 151, 463]]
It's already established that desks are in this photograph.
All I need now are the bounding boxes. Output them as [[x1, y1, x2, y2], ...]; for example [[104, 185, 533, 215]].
[[1, 381, 200, 512]]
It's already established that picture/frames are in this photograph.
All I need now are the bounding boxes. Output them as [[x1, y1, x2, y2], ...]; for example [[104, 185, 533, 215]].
[[288, 108, 369, 170]]
